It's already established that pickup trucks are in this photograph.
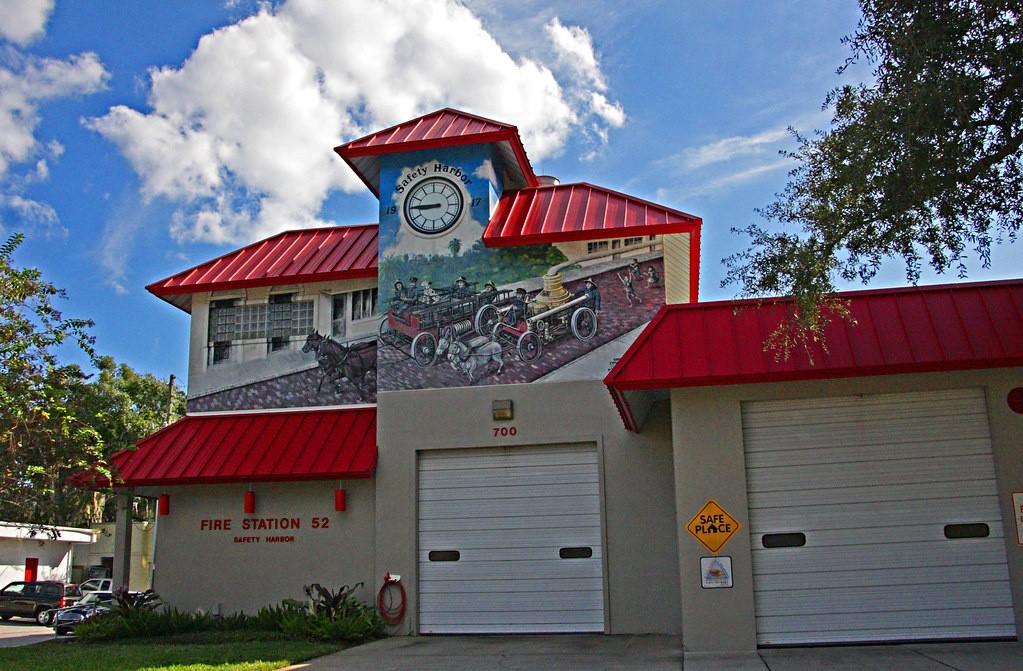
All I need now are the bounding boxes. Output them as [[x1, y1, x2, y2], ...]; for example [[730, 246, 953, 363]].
[[77, 579, 142, 593]]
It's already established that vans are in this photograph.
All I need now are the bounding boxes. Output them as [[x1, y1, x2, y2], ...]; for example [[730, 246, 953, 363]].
[[0, 581, 82, 625]]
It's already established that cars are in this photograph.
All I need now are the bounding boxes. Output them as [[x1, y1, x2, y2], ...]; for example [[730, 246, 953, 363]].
[[52, 593, 153, 635]]
